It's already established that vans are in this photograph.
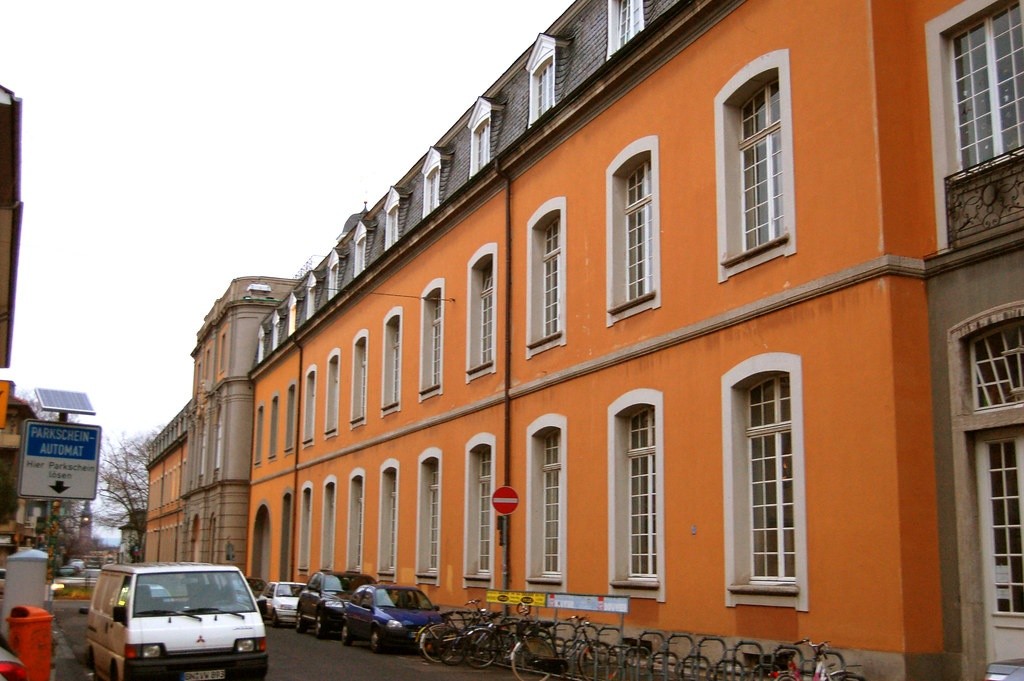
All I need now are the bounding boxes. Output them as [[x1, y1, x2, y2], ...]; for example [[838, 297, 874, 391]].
[[83, 563, 269, 681]]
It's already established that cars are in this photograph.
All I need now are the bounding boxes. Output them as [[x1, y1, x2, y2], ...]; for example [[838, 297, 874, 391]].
[[340, 585, 443, 653], [57, 559, 85, 577], [259, 582, 308, 627]]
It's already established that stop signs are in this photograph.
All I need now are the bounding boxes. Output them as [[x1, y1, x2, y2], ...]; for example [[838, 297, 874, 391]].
[[491, 487, 518, 516]]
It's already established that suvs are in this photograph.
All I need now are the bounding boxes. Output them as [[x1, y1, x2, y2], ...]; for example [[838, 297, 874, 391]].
[[295, 571, 378, 639]]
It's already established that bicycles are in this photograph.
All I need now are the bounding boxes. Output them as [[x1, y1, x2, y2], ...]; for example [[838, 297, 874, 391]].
[[767, 638, 855, 681], [415, 599, 535, 669], [510, 615, 619, 680]]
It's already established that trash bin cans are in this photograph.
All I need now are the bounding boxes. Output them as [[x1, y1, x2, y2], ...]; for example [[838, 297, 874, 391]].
[[6, 605, 54, 681]]
